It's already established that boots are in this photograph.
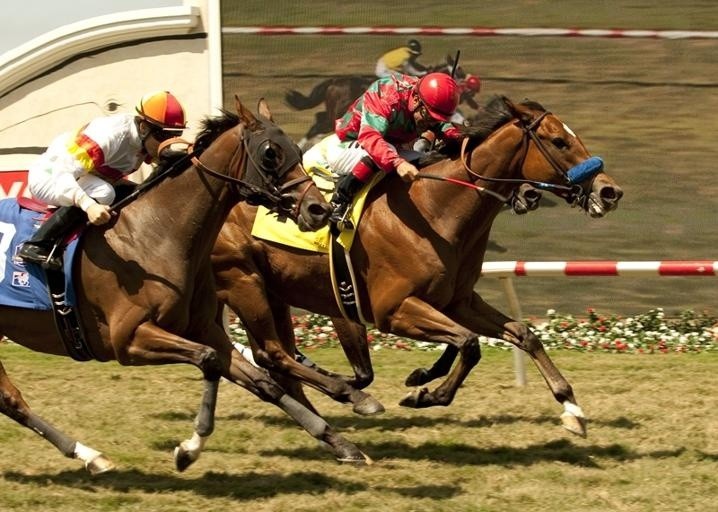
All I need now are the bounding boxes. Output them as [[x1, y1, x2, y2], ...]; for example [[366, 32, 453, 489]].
[[323, 154, 379, 231], [19, 196, 102, 268]]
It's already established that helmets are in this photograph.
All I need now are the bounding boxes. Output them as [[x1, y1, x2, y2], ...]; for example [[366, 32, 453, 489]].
[[404, 39, 422, 55], [134, 89, 191, 133], [410, 71, 462, 124], [464, 74, 481, 94]]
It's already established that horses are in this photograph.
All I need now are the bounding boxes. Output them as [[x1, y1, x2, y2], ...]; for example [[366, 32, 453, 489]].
[[0, 91, 377, 478], [290, 181, 542, 410], [282, 54, 478, 152], [213, 91, 625, 442]]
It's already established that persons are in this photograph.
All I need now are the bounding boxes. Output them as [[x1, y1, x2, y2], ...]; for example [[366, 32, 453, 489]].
[[322, 72, 459, 229], [449, 75, 482, 127], [376, 40, 428, 78], [19, 90, 187, 269]]
